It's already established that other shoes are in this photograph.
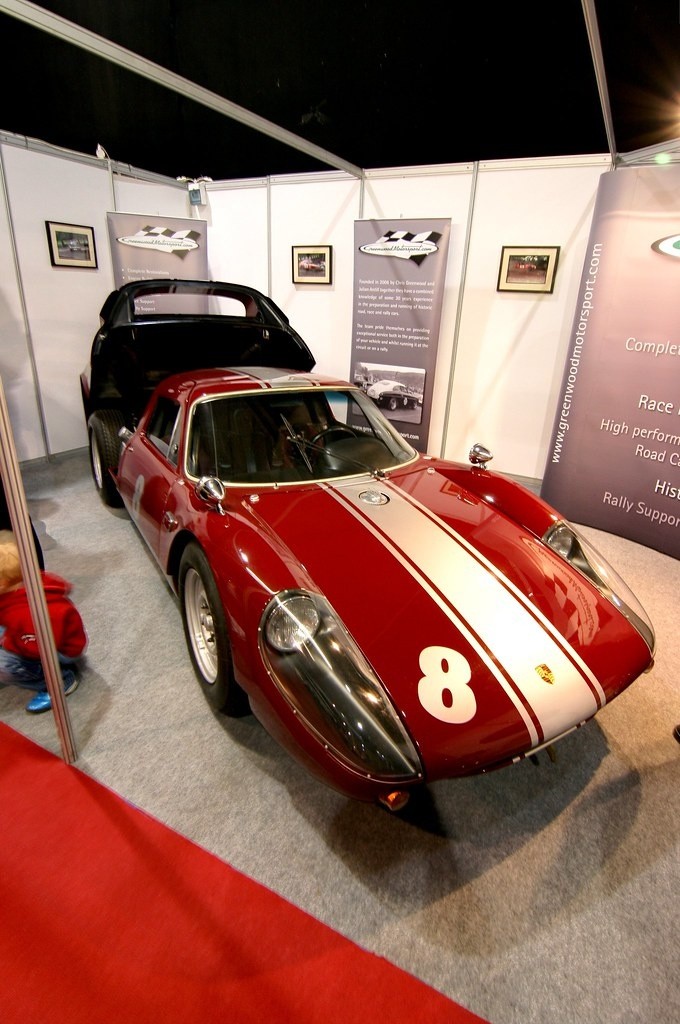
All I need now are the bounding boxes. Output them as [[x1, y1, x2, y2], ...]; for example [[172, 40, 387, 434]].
[[26, 679, 77, 714], [673, 725, 680, 744]]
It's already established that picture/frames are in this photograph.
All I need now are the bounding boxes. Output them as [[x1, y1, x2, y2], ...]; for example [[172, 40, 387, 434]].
[[45, 220, 98, 269], [495, 245, 561, 293], [292, 245, 333, 284]]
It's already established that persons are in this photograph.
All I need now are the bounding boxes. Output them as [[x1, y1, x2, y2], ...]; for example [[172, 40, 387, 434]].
[[0, 529, 89, 713]]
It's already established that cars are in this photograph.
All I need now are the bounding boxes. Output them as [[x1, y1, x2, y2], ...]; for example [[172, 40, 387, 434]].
[[366, 380, 418, 410], [517, 261, 536, 271], [79, 278, 654, 811], [69, 242, 81, 252]]
